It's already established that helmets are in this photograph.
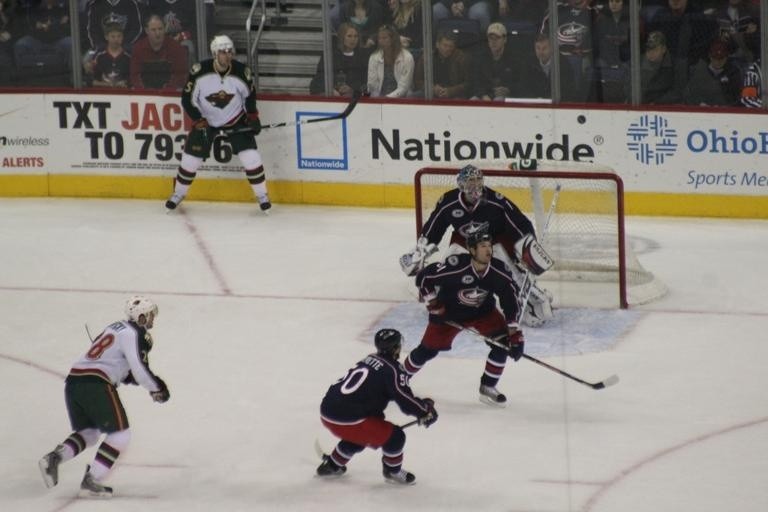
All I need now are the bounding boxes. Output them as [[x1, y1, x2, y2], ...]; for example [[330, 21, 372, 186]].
[[209, 35, 234, 58], [469, 234, 493, 243], [375, 330, 402, 354], [456, 166, 485, 194], [125, 297, 160, 323]]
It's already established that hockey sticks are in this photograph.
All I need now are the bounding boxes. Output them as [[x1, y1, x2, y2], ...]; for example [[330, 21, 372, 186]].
[[314, 413, 433, 459], [208, 89, 362, 134], [411, 291, 620, 390], [83, 323, 160, 401]]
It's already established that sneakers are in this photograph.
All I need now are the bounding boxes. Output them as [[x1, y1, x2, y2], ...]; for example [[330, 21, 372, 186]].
[[383, 468, 415, 483], [81, 465, 113, 493], [166, 194, 184, 208], [325, 458, 347, 474], [480, 385, 506, 403], [45, 452, 59, 485], [258, 194, 272, 210]]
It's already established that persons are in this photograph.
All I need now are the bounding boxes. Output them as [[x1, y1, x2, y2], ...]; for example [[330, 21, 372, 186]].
[[402, 232, 525, 404], [315, 328, 439, 485], [39, 292, 170, 493], [400, 164, 556, 328], [0, 0, 215, 94], [164, 34, 272, 212], [312, 0, 761, 111]]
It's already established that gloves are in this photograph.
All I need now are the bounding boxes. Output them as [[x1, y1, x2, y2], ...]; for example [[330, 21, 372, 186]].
[[150, 377, 170, 402], [504, 330, 524, 361], [429, 307, 445, 321], [400, 252, 421, 276], [248, 119, 261, 134], [417, 398, 438, 426], [192, 119, 208, 128]]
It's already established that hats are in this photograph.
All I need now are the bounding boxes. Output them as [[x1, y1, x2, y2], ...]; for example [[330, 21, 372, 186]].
[[710, 42, 728, 57], [487, 23, 506, 37], [647, 31, 664, 47]]
